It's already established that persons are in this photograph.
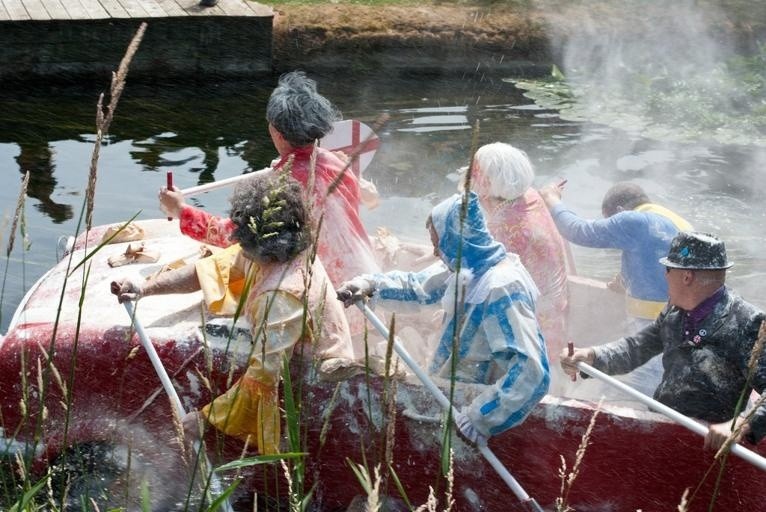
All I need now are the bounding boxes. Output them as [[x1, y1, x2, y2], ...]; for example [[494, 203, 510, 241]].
[[559, 230, 765, 455], [540, 178, 691, 399], [156, 67, 379, 320], [332, 191, 553, 447], [397, 142, 581, 366], [111, 164, 357, 457]]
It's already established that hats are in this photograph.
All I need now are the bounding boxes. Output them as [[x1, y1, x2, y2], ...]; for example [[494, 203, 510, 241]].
[[658, 230, 735, 270]]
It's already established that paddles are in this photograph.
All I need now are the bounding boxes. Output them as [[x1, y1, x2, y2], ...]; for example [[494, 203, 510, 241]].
[[167, 120, 381, 221]]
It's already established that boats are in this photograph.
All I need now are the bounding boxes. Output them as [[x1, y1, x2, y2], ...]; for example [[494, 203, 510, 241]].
[[0, 216, 766, 512]]
